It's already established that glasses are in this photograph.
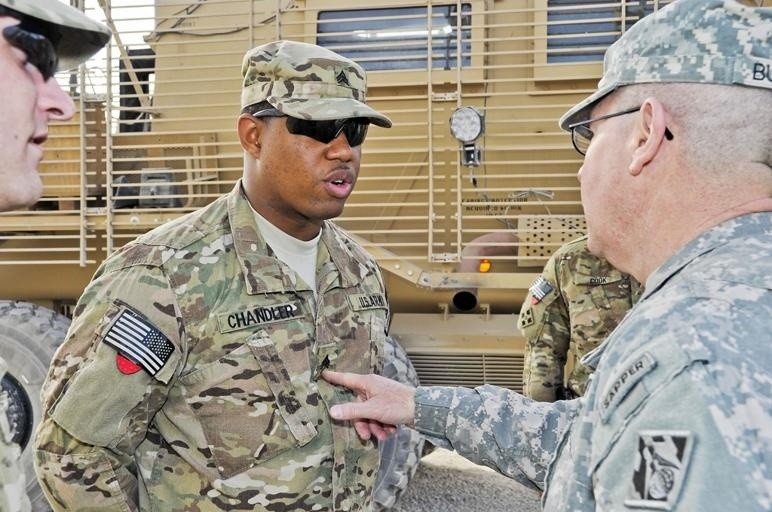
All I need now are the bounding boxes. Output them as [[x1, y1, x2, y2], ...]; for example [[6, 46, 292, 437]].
[[2, 21, 59, 81], [568, 105, 674, 156], [251, 107, 370, 147]]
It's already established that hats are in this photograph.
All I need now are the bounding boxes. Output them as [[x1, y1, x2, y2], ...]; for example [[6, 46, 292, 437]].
[[558, 0, 772, 133], [0, 0, 113, 72], [240, 39, 393, 128]]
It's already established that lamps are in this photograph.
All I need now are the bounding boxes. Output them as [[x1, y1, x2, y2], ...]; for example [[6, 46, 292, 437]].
[[448, 106, 486, 187]]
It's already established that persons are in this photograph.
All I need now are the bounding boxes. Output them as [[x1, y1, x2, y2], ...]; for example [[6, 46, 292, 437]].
[[1, 0, 112, 212], [319, 0, 772, 512], [32, 39, 392, 512], [516, 232, 648, 403]]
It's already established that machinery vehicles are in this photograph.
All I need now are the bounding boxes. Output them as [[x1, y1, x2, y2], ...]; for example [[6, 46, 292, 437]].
[[1, 2, 772, 511]]
